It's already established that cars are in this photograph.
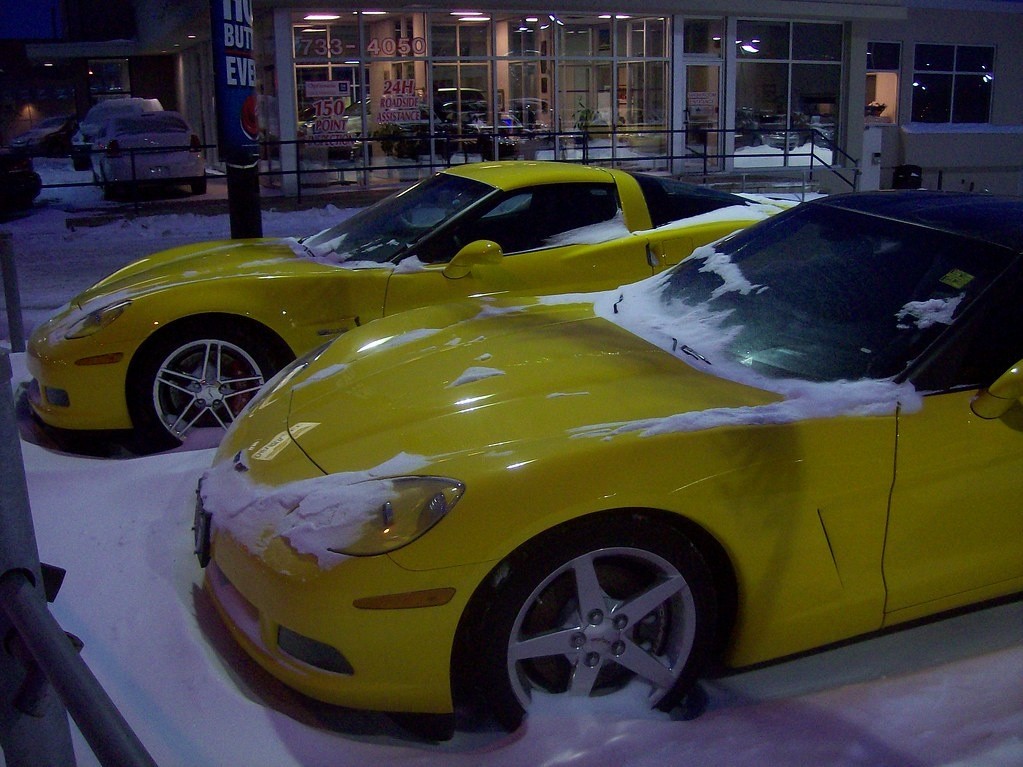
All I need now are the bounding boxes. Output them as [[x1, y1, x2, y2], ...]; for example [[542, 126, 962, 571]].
[[26, 159, 803, 450], [71, 97, 165, 171], [91, 111, 210, 196], [298, 86, 485, 160], [8, 113, 83, 159], [735, 106, 835, 153], [192, 191, 1023, 731], [463, 98, 551, 161]]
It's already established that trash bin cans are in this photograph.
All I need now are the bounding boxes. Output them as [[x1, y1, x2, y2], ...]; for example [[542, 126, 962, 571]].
[[892, 163, 923, 191]]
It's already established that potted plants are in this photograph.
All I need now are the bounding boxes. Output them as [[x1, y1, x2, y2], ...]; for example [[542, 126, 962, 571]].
[[570, 98, 600, 150], [380, 117, 422, 182]]
